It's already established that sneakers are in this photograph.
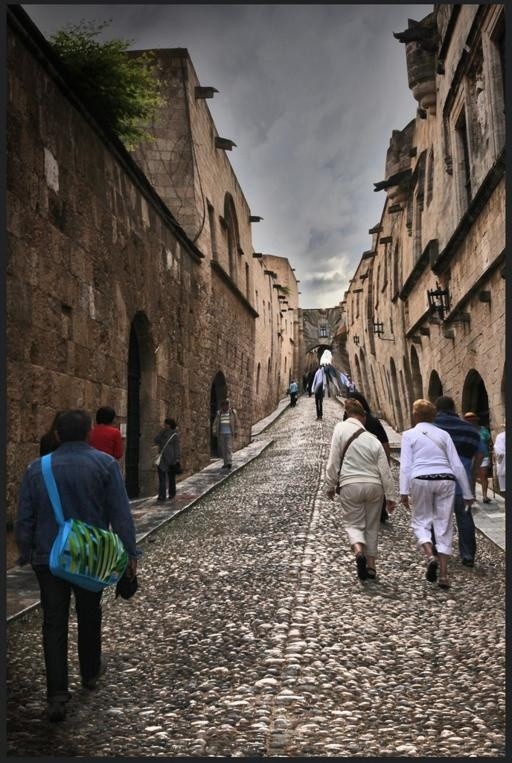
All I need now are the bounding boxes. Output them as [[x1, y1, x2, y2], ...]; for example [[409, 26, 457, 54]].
[[169, 491, 176, 499], [461, 558, 475, 568], [79, 653, 109, 689], [47, 690, 72, 705], [156, 496, 166, 505], [483, 497, 491, 503], [380, 512, 389, 523], [221, 463, 232, 470]]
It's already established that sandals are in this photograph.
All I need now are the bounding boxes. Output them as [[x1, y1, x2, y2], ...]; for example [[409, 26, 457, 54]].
[[438, 578, 451, 589], [356, 553, 366, 580], [426, 558, 438, 582], [366, 566, 377, 579]]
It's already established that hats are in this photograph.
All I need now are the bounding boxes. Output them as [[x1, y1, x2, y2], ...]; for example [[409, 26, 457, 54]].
[[463, 411, 480, 422], [114, 571, 138, 600]]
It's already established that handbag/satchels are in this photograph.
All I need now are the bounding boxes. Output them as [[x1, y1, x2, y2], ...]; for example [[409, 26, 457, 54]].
[[48, 518, 128, 593], [212, 423, 218, 437], [169, 462, 182, 474], [414, 426, 446, 452], [152, 445, 162, 467], [336, 478, 342, 495]]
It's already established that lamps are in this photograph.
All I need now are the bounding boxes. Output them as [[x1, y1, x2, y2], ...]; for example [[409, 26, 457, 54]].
[[427, 281, 470, 324], [353, 335, 360, 347], [373, 317, 395, 345]]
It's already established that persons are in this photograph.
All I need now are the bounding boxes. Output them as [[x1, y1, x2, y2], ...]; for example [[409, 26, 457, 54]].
[[288, 362, 356, 421], [464, 412, 490, 504], [153, 418, 183, 501], [212, 398, 241, 471], [90, 406, 124, 458], [15, 409, 139, 728], [39, 411, 64, 456], [325, 398, 398, 581], [431, 395, 482, 567], [399, 398, 474, 587], [493, 423, 506, 494], [343, 392, 391, 523]]
